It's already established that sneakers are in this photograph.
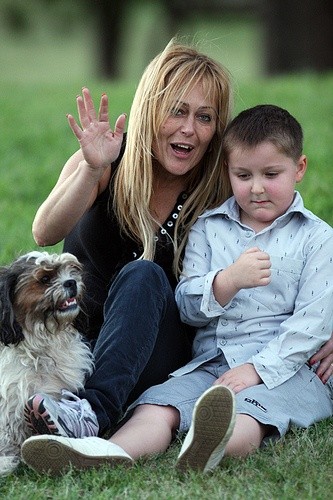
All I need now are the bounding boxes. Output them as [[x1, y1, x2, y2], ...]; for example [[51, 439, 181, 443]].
[[176, 385, 237, 487], [21, 433, 135, 479], [25, 393, 98, 439]]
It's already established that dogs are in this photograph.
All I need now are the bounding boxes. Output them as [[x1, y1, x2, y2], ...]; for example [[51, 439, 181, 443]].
[[0, 251, 96, 481]]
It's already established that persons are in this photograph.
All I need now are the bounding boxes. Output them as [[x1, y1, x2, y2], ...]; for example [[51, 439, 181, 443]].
[[21, 102, 333, 473], [24, 46, 333, 451]]
